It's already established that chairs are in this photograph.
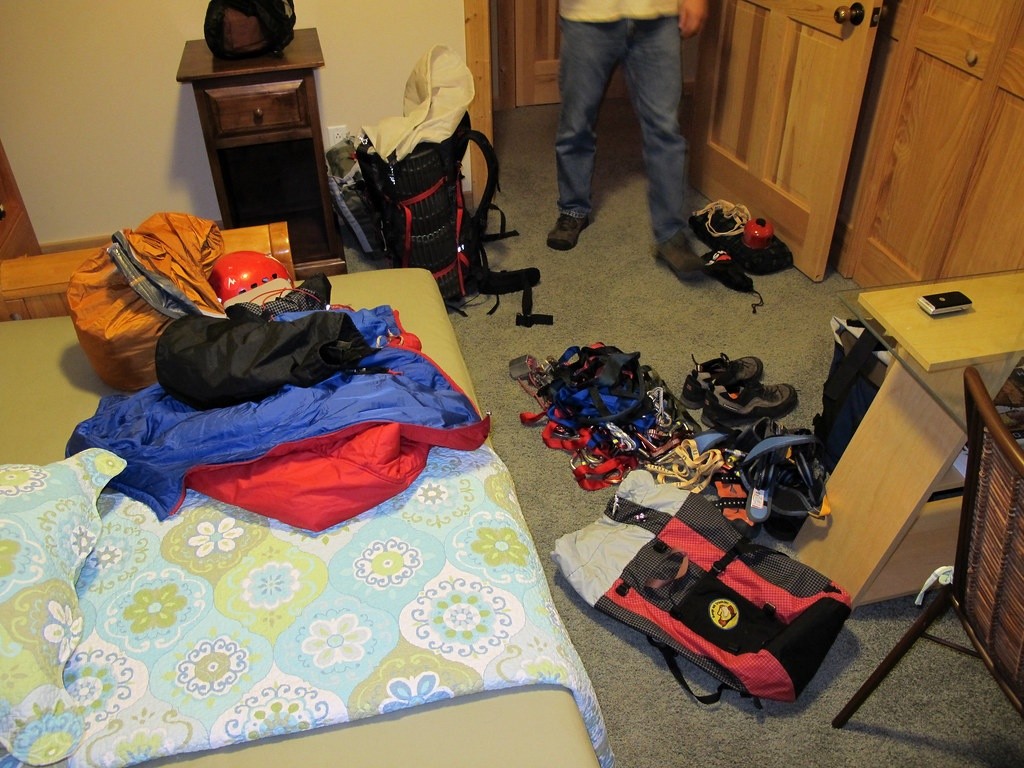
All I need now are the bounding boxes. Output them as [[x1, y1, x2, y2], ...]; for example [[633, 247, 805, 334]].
[[831, 367, 1023, 728]]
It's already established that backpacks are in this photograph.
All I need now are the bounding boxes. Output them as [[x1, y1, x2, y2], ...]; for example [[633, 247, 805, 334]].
[[549, 469, 851, 706], [355, 111, 554, 327]]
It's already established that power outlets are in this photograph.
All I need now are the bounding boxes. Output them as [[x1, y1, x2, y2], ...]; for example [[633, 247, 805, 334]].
[[328, 126, 346, 148]]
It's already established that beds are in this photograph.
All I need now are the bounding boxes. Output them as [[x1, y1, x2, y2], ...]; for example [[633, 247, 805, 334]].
[[1, 268, 615, 768]]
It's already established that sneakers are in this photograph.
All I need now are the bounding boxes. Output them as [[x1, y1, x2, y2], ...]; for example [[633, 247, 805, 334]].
[[679, 353, 763, 410], [546, 214, 589, 250], [702, 379, 796, 428], [655, 241, 706, 282]]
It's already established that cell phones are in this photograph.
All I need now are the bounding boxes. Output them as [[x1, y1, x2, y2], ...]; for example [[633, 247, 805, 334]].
[[918, 291, 972, 315]]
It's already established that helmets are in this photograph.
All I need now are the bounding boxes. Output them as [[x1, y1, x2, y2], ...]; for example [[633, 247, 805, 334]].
[[209, 251, 295, 309]]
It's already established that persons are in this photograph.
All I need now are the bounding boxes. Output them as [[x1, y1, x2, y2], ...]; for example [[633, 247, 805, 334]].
[[547, 0, 707, 281]]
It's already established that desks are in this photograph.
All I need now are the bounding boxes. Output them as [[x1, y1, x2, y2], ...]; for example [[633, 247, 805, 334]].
[[790, 267, 1024, 610]]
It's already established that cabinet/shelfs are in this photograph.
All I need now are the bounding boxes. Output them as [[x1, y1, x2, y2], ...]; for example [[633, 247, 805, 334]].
[[0, 139, 42, 260], [176, 28, 347, 281]]
